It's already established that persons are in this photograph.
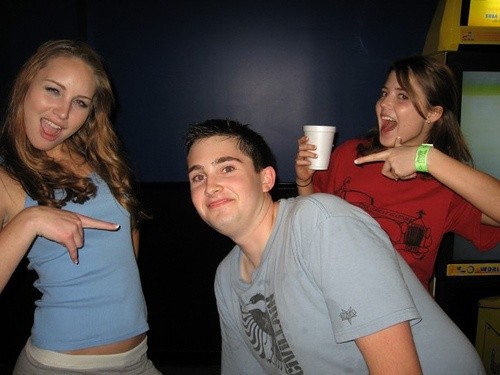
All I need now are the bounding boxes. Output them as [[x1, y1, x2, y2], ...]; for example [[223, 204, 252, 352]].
[[0, 40, 163, 375], [294, 57, 500, 293], [186, 119, 487, 375]]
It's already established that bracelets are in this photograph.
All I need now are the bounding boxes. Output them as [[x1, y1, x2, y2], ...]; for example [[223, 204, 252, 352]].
[[415, 144, 434, 172], [296, 180, 311, 188]]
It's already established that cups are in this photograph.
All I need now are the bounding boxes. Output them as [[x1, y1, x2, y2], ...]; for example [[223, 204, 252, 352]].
[[302, 124, 337, 171]]
